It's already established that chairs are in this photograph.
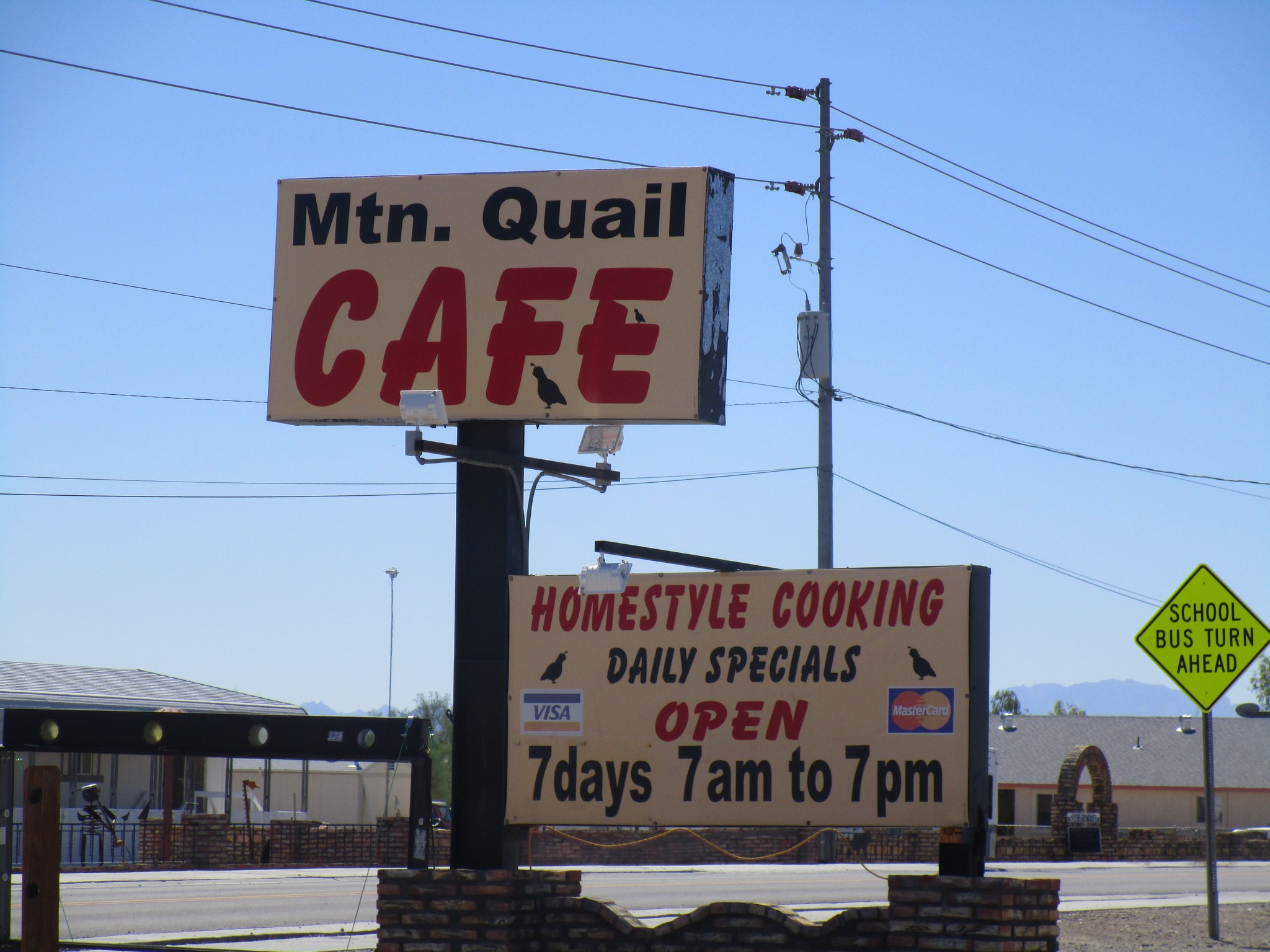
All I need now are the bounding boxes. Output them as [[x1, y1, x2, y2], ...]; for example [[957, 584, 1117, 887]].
[[845, 830, 873, 861]]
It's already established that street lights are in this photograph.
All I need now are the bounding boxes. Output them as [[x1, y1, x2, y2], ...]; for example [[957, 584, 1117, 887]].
[[385, 567, 398, 717]]
